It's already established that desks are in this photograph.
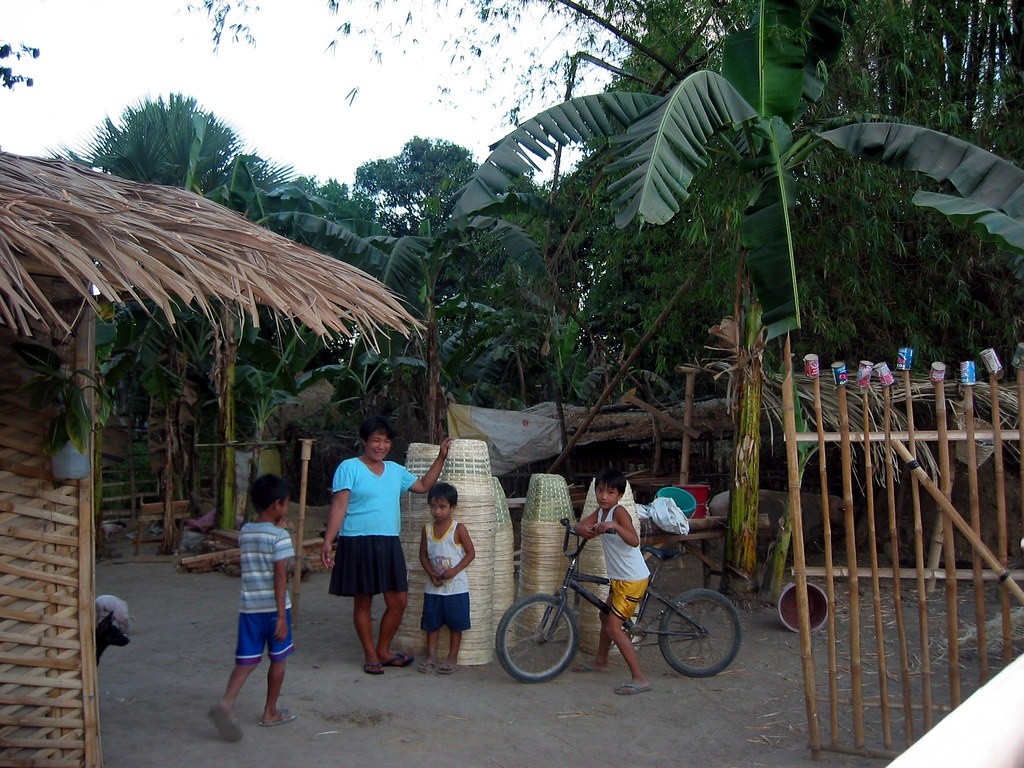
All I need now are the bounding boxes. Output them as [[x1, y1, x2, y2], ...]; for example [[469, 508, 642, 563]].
[[638, 527, 771, 609]]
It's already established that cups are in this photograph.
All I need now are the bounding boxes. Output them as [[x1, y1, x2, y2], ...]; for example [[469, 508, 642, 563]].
[[803, 354, 819, 379], [896, 347, 913, 371], [831, 362, 848, 387], [856, 361, 874, 388], [929, 362, 946, 382], [979, 348, 1002, 375], [873, 362, 894, 386], [960, 361, 976, 385], [1011, 343, 1024, 370]]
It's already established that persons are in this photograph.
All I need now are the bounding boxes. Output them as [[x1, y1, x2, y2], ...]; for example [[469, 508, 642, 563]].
[[418, 483, 475, 674], [572, 469, 652, 694], [209, 473, 298, 743], [320, 417, 455, 675]]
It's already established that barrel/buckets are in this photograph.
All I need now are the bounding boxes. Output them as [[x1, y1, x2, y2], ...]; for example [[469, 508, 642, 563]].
[[673, 485, 710, 519], [778, 581, 829, 633], [229, 448, 283, 531], [655, 487, 696, 519]]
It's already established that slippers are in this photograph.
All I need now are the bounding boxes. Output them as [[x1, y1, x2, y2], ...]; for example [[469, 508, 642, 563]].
[[615, 683, 650, 695], [419, 662, 435, 672], [364, 663, 384, 675], [437, 663, 460, 674], [209, 704, 243, 742], [259, 709, 297, 726], [382, 653, 414, 666], [573, 661, 609, 673]]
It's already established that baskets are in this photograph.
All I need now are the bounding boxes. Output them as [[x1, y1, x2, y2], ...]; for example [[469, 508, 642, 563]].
[[369, 437, 644, 667]]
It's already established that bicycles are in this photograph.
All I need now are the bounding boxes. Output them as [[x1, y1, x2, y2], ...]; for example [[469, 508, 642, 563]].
[[495, 518, 741, 683]]
